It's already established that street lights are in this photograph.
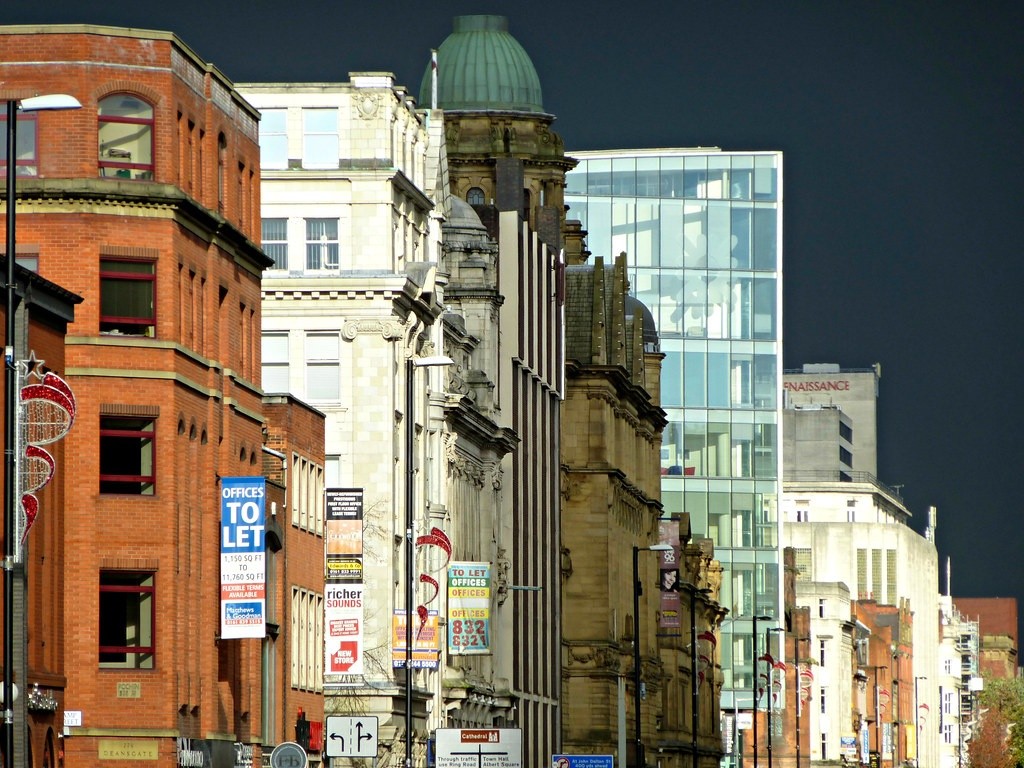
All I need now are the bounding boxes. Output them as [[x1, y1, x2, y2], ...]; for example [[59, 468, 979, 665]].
[[753, 616, 771, 768], [690, 589, 713, 768], [767, 628, 784, 768], [915, 677, 927, 768], [875, 666, 888, 768], [407, 357, 454, 768], [795, 638, 804, 768], [7, 94, 81, 768], [633, 544, 674, 768]]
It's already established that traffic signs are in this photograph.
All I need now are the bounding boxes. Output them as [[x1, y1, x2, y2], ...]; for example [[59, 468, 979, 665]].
[[436, 728, 523, 768]]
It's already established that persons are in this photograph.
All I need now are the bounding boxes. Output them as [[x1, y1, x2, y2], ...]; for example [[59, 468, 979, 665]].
[[661, 570, 677, 591]]
